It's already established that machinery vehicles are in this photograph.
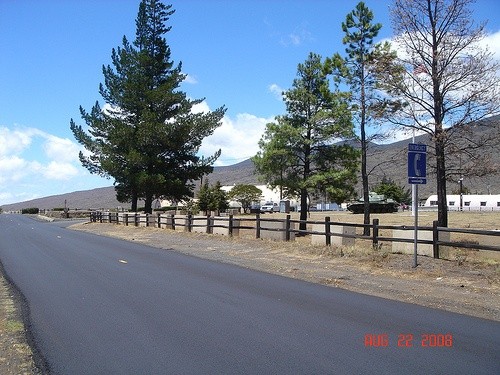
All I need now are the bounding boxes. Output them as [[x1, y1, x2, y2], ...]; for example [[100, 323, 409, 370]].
[[346, 191, 400, 214]]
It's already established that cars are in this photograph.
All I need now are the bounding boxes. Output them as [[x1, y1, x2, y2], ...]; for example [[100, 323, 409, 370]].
[[259, 202, 280, 213]]
